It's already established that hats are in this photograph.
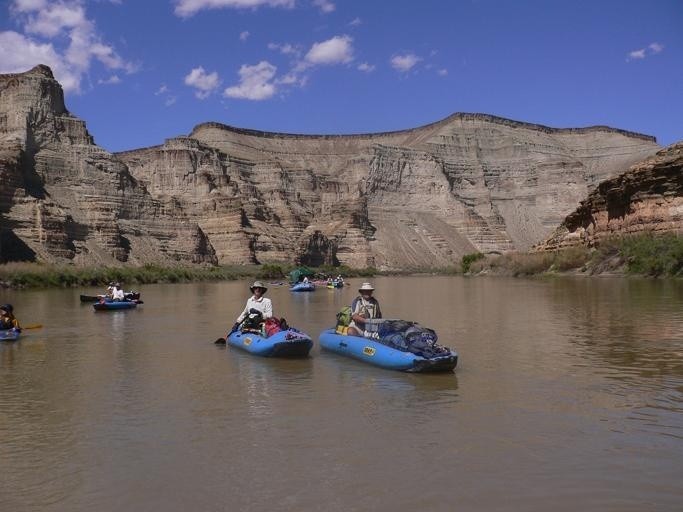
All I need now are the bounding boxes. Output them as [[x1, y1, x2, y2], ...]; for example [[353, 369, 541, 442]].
[[116, 283, 120, 287], [359, 282, 375, 290], [110, 282, 114, 285], [250, 281, 267, 294]]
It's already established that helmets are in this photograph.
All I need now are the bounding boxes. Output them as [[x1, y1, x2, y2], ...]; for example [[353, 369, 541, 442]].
[[1, 304, 13, 313]]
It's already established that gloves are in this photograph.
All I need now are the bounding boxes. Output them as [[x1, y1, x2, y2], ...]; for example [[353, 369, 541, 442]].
[[232, 322, 240, 332], [250, 308, 262, 315]]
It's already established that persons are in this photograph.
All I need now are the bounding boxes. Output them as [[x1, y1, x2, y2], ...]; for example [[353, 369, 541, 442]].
[[0, 304, 14, 328], [303, 275, 308, 284], [106, 281, 124, 302], [328, 274, 332, 282], [347, 282, 382, 335], [232, 280, 272, 331], [336, 274, 343, 281]]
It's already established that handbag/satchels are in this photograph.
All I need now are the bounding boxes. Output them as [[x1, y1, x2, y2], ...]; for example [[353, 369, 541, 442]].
[[265, 320, 280, 336], [391, 320, 409, 332]]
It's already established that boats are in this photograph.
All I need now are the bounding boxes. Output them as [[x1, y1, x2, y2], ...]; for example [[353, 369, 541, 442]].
[[227, 327, 458, 372], [0, 327, 19, 341], [80, 292, 144, 310], [289, 281, 343, 292]]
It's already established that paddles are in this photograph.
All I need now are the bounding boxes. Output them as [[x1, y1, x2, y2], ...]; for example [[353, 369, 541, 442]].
[[214, 312, 250, 345], [11, 323, 43, 331]]
